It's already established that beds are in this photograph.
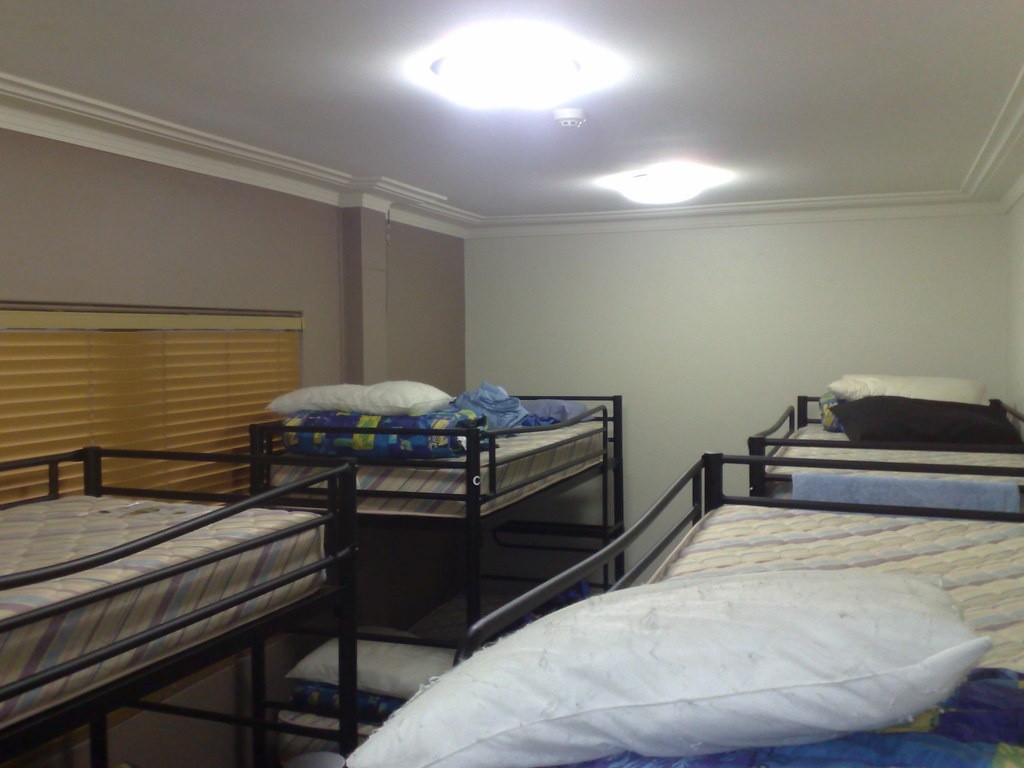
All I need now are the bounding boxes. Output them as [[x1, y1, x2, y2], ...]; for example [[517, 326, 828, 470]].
[[250, 394, 626, 768], [749, 394, 1024, 496], [1, 445, 358, 768], [454, 453, 1024, 768]]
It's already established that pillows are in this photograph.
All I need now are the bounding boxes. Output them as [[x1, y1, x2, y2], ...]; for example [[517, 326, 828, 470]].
[[349, 571, 992, 768], [828, 396, 1022, 447], [266, 381, 457, 416], [829, 374, 980, 404], [284, 637, 457, 698]]
